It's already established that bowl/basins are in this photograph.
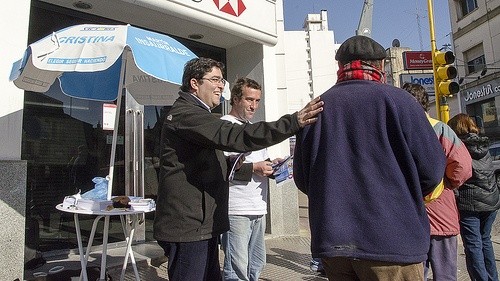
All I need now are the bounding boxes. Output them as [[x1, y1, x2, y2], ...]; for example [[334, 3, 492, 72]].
[[61, 195, 155, 212]]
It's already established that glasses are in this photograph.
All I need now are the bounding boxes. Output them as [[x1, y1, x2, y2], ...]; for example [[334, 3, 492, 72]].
[[197, 78, 225, 87]]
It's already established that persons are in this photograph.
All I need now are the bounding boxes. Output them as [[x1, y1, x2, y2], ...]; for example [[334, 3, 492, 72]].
[[154, 58, 324, 281], [293, 36, 446, 281], [219, 77, 284, 281], [447, 113, 500, 281], [26, 144, 97, 227], [403, 83, 472, 281]]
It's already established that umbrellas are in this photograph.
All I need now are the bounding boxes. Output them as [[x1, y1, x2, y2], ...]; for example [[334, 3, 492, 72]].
[[10, 25, 231, 281]]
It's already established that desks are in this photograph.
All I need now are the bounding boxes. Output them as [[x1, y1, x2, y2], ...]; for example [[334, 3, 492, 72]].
[[55, 203, 157, 281]]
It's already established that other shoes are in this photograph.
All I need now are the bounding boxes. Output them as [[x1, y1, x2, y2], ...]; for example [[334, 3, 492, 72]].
[[310, 262, 323, 270]]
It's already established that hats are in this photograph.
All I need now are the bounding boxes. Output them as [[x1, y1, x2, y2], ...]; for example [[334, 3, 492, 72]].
[[335, 36, 386, 60]]
